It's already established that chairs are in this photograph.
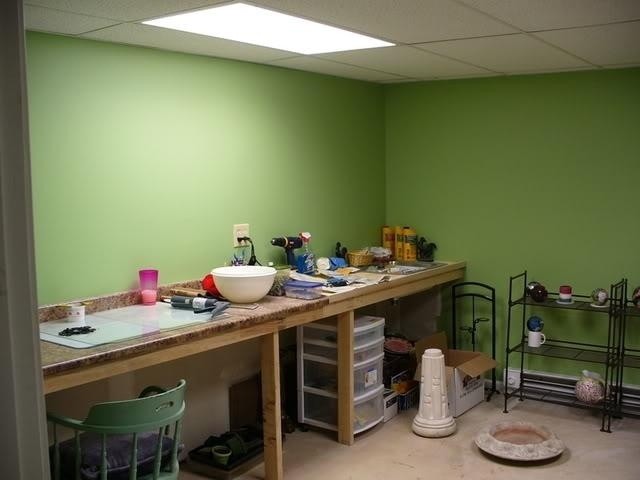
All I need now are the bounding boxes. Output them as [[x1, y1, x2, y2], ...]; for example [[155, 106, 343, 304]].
[[47, 380, 187, 480]]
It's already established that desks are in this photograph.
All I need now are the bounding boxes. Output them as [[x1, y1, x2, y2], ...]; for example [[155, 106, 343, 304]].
[[39, 263, 465, 480]]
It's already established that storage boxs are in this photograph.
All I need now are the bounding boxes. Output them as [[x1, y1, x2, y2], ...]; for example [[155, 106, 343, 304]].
[[414, 335, 496, 416]]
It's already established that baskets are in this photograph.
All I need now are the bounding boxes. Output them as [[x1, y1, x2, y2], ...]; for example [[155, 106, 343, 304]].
[[348, 249, 374, 266]]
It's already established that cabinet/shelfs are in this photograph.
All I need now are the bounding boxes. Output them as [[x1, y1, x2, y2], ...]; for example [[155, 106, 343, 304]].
[[296, 316, 386, 434], [504, 271, 640, 432]]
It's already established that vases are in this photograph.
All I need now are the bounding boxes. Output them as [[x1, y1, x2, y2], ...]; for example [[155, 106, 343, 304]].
[[227, 432, 246, 456]]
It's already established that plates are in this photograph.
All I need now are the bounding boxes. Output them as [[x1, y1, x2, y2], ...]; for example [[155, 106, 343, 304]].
[[556, 299, 575, 304], [590, 303, 609, 308]]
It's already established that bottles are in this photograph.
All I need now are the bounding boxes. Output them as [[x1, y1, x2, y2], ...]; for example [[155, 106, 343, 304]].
[[382, 225, 416, 262]]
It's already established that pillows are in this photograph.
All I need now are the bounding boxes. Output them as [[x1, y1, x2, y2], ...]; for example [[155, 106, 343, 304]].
[[55, 434, 184, 478]]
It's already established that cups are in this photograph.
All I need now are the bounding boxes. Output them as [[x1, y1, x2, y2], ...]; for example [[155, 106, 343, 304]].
[[139, 269, 159, 305], [559, 286, 572, 302], [66, 304, 85, 322], [528, 332, 546, 348]]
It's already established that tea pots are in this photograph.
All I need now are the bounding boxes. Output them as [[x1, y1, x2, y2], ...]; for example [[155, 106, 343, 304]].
[[575, 370, 610, 403]]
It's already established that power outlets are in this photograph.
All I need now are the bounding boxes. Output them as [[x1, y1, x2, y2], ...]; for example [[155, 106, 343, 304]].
[[234, 225, 250, 247]]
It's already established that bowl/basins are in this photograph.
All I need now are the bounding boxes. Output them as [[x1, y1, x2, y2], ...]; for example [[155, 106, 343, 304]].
[[211, 266, 277, 303]]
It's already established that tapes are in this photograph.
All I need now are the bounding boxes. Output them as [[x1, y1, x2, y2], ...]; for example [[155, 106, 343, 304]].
[[212, 446, 232, 457]]
